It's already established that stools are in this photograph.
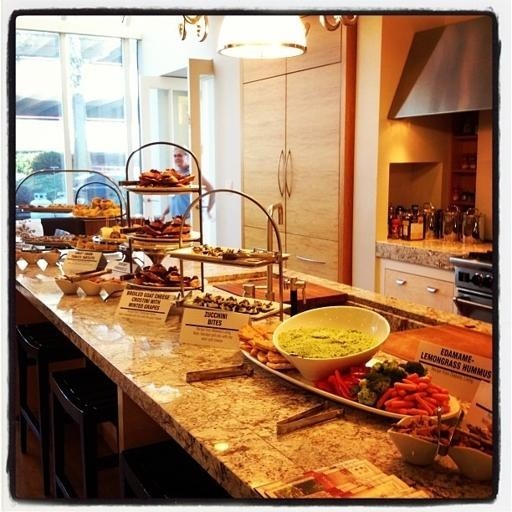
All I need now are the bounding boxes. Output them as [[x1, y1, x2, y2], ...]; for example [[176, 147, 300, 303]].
[[15, 321, 235, 500]]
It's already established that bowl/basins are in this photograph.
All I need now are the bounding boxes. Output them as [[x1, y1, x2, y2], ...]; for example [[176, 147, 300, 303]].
[[387, 415, 449, 466], [56, 280, 79, 295], [447, 429, 493, 483], [74, 278, 105, 297], [103, 280, 128, 297], [272, 305, 391, 381], [22, 251, 45, 265], [42, 251, 62, 265]]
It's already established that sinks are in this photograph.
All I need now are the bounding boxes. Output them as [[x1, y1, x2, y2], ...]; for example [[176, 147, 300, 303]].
[[284, 294, 436, 333]]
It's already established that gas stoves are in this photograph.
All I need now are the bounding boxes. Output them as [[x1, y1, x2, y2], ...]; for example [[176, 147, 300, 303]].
[[450, 249, 493, 269]]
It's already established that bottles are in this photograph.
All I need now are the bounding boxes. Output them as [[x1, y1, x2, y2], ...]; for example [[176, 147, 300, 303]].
[[388, 205, 485, 243]]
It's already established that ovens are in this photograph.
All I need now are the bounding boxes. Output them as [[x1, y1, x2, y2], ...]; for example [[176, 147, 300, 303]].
[[454, 266, 493, 324]]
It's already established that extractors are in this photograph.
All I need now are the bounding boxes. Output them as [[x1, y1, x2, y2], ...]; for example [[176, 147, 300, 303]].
[[387, 16, 492, 119]]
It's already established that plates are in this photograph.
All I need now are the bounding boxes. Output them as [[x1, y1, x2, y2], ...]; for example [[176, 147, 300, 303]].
[[239, 323, 461, 422]]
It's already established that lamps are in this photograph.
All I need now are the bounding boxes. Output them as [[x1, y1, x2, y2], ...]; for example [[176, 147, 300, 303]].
[[215, 16, 307, 60]]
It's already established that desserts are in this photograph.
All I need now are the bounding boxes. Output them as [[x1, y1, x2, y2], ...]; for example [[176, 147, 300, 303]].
[[72, 168, 294, 371]]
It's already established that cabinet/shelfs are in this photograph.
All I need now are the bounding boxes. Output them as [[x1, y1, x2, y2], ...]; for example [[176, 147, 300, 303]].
[[378, 259, 460, 315], [120, 141, 291, 322], [449, 135, 478, 207], [241, 15, 358, 285]]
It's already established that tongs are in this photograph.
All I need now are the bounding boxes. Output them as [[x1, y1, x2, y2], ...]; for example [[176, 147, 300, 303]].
[[435, 406, 465, 457], [186, 362, 254, 382], [71, 268, 113, 284], [277, 401, 345, 434]]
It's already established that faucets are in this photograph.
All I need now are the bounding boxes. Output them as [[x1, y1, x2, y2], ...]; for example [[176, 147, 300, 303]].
[[267, 202, 284, 292]]
